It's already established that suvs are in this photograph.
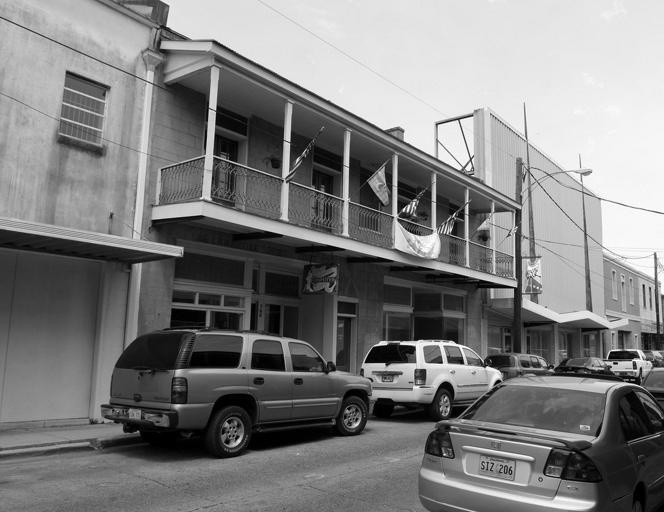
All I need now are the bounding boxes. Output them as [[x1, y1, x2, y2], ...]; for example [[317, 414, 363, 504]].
[[358, 336, 506, 420], [99, 328, 374, 456]]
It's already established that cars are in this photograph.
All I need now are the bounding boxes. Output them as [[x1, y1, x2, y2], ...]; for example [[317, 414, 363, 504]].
[[416, 373, 663, 511], [482, 343, 663, 412]]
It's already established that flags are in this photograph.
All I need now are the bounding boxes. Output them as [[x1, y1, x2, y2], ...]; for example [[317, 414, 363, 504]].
[[523, 256, 542, 293], [366, 164, 391, 207], [506, 225, 520, 236], [401, 185, 426, 218], [284, 131, 320, 184], [478, 214, 489, 230], [437, 208, 460, 235]]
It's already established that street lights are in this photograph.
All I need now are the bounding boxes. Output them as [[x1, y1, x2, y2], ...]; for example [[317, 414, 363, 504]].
[[514, 155, 593, 353]]
